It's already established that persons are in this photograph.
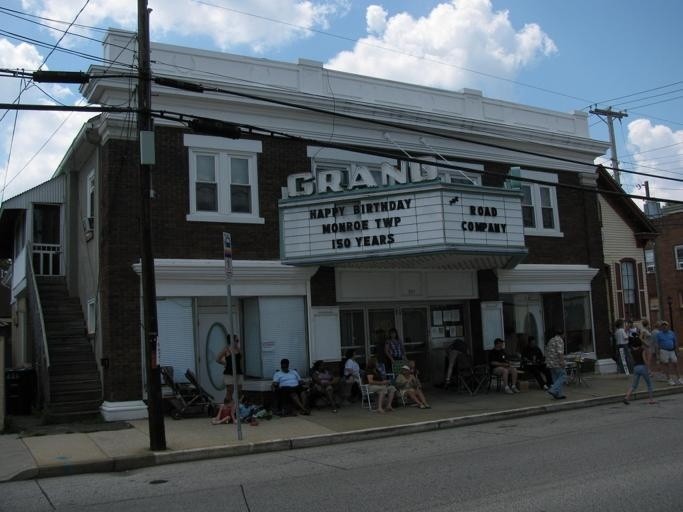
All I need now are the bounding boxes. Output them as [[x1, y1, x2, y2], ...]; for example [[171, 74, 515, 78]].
[[447, 328, 567, 400], [212, 394, 273, 426], [271, 327, 432, 416], [215, 334, 244, 404], [611, 319, 679, 405]]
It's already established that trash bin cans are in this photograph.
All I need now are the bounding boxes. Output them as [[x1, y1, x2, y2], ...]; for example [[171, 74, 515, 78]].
[[4, 367, 35, 415]]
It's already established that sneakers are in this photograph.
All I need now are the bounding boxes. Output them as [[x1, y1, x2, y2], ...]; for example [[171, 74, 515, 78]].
[[418, 403, 430, 409], [677, 378, 682, 384], [667, 378, 675, 386], [504, 386, 514, 393], [512, 386, 520, 393], [649, 399, 656, 403], [623, 396, 629, 404]]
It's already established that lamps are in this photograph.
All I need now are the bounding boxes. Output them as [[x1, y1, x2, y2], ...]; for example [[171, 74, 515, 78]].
[[84, 230, 93, 242]]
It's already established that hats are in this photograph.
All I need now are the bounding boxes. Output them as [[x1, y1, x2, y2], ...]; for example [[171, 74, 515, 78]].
[[401, 365, 410, 371]]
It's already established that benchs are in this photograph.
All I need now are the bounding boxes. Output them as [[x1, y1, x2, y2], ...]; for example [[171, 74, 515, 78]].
[[311, 360, 345, 390]]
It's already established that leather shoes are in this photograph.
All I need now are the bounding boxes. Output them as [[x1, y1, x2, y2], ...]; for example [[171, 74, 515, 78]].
[[299, 407, 310, 415]]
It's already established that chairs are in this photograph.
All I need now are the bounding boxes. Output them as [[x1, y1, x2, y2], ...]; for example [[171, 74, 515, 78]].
[[356, 374, 376, 410], [274, 369, 301, 414], [459, 350, 589, 393], [394, 362, 419, 405]]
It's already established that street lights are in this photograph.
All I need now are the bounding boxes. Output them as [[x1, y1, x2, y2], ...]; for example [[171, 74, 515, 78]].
[[667, 295, 674, 338]]
